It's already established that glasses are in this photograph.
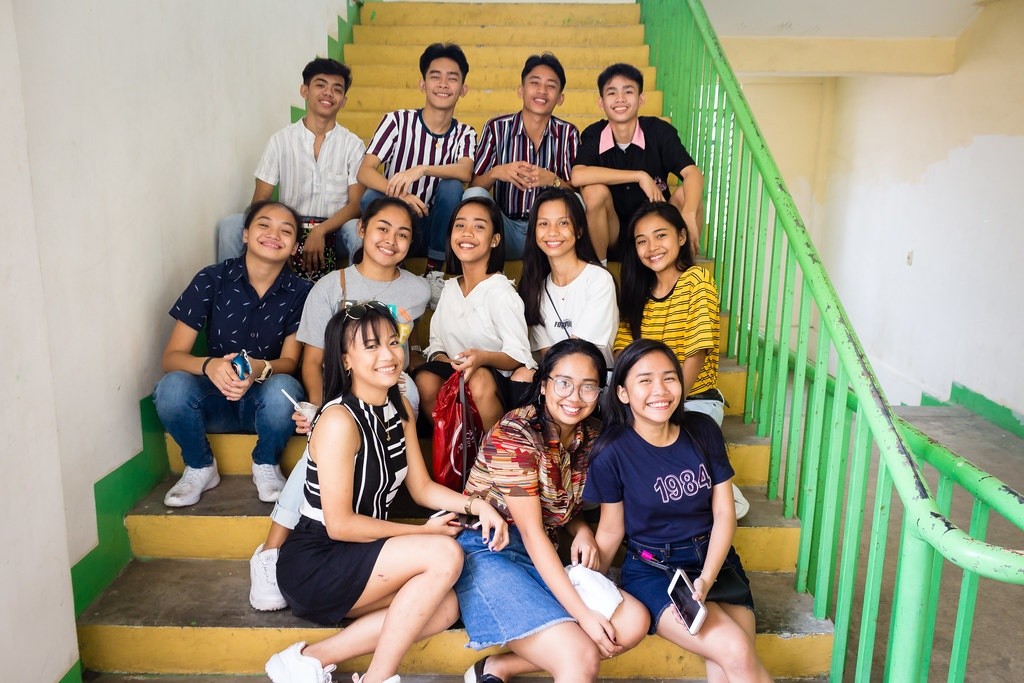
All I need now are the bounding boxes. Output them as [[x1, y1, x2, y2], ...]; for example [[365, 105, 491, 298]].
[[548, 375, 603, 402], [343, 300, 386, 323]]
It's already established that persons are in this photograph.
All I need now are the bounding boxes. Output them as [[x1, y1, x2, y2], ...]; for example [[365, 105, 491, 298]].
[[153, 201, 313, 507], [412, 197, 538, 435], [249, 196, 432, 611], [518, 186, 621, 396], [455, 337, 651, 683], [613, 200, 749, 520], [462, 55, 579, 261], [582, 338, 773, 683], [570, 63, 703, 269], [218, 59, 367, 273], [357, 42, 477, 311], [265, 300, 508, 683]]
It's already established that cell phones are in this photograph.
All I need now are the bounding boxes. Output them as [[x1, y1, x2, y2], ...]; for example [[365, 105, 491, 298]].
[[429, 511, 482, 529], [667, 569, 708, 636], [232, 348, 252, 381]]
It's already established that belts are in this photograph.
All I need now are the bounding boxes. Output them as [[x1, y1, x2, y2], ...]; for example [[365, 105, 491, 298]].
[[686, 388, 731, 408]]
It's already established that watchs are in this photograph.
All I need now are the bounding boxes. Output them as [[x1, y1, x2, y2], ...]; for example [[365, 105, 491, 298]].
[[553, 176, 561, 188], [255, 360, 273, 384]]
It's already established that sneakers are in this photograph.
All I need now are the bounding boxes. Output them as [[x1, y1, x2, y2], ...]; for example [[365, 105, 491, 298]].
[[164, 457, 220, 507], [265, 639, 336, 683], [421, 271, 445, 310], [248, 543, 288, 611], [351, 671, 401, 683], [252, 462, 288, 502]]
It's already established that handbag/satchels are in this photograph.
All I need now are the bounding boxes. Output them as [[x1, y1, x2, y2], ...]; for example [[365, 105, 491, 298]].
[[431, 370, 484, 492]]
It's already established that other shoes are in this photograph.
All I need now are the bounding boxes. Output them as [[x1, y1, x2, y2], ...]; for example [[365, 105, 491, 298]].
[[464, 655, 504, 683], [733, 496, 750, 519]]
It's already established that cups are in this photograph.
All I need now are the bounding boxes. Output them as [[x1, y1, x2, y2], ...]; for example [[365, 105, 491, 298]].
[[294, 401, 318, 435]]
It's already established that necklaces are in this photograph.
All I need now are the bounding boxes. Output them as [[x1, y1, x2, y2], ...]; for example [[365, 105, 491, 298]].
[[553, 283, 570, 300], [421, 111, 451, 148], [364, 401, 391, 441], [356, 266, 394, 300]]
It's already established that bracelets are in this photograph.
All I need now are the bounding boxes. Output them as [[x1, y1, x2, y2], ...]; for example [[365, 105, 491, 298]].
[[409, 345, 421, 352], [202, 357, 214, 374], [431, 354, 447, 361], [464, 494, 482, 515]]
